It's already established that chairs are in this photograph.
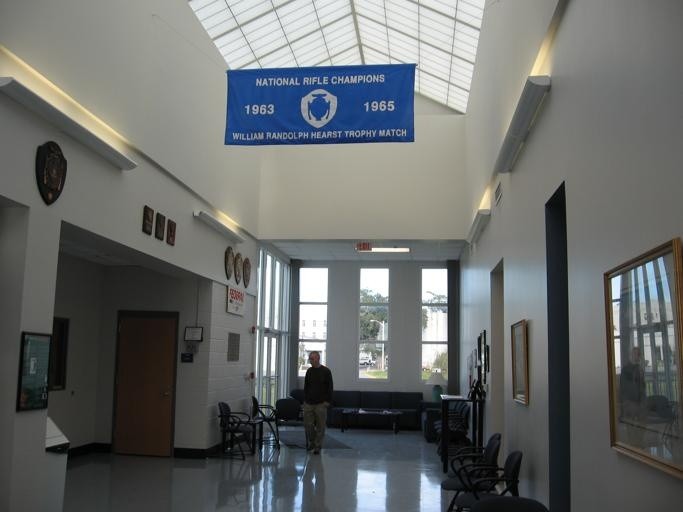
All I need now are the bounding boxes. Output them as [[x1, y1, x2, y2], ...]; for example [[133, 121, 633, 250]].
[[218, 401, 254, 461], [252, 395, 280, 450], [420, 399, 549, 512]]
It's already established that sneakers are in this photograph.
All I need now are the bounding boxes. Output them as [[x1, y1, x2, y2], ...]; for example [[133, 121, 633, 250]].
[[307, 447, 321, 454]]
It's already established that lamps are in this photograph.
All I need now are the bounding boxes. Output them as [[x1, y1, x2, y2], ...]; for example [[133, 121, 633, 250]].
[[491, 75, 552, 176], [465, 209, 491, 244], [355, 247, 411, 253], [0, 76, 138, 170], [193, 210, 246, 244]]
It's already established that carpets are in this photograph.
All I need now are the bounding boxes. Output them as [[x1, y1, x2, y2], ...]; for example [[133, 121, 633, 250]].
[[278, 431, 352, 449]]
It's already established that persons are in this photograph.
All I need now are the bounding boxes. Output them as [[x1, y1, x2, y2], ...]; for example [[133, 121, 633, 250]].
[[302, 350, 332, 456], [620, 347, 648, 435], [301, 451, 326, 512]]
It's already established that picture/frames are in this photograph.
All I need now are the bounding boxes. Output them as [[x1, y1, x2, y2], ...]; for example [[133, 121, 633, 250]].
[[480, 329, 485, 384]]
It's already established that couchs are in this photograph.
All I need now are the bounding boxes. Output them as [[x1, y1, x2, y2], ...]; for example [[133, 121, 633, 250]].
[[275, 389, 423, 431]]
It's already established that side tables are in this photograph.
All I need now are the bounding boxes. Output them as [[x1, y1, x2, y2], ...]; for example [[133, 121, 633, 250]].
[[228, 420, 264, 453]]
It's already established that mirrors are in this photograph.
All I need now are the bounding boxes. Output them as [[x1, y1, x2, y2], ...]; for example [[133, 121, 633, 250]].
[[604, 237, 683, 482], [510, 319, 529, 406]]
